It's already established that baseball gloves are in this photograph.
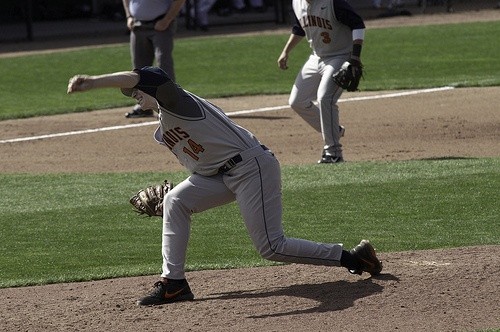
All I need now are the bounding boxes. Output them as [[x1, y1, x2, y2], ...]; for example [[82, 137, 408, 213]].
[[128, 179, 174, 217], [330, 57, 366, 93]]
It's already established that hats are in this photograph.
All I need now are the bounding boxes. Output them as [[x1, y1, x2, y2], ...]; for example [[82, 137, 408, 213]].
[[120, 66, 170, 98]]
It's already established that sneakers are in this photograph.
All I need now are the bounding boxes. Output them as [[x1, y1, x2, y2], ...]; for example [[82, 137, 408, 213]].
[[140, 277, 194, 306], [339, 126, 345, 140], [347, 239, 383, 276], [317, 155, 343, 163]]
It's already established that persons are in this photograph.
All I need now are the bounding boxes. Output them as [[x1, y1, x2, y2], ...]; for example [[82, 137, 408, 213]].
[[123, 0, 185, 118], [114, 0, 126, 21], [67, 66, 383, 306], [177, 0, 290, 32], [373, 0, 451, 9], [278, 0, 366, 163]]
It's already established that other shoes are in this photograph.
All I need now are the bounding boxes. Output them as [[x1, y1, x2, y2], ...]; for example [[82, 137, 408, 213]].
[[125, 103, 153, 118]]
